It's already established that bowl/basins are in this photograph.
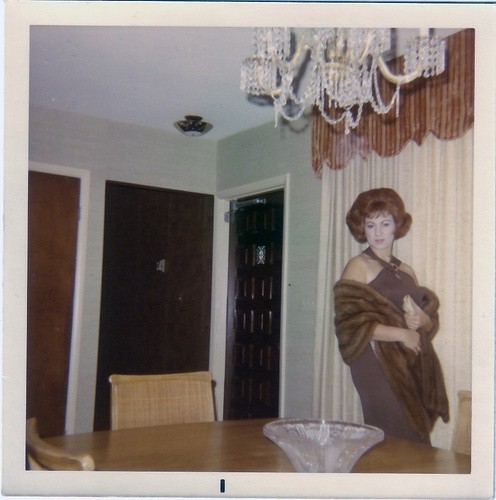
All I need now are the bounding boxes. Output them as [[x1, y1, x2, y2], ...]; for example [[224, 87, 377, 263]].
[[261, 417, 385, 473]]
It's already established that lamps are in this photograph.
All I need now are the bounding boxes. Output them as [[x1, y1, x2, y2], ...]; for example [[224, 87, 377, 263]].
[[172, 115, 213, 137], [239, 27, 447, 134]]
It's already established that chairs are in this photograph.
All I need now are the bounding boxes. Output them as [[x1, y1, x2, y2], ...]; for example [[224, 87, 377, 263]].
[[109, 371, 216, 431], [25, 417, 97, 470]]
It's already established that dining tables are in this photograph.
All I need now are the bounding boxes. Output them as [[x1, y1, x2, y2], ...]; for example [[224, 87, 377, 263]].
[[38, 416, 471, 473]]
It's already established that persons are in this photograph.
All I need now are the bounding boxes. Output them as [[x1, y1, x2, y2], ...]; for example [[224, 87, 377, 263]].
[[334, 188, 450, 448]]
